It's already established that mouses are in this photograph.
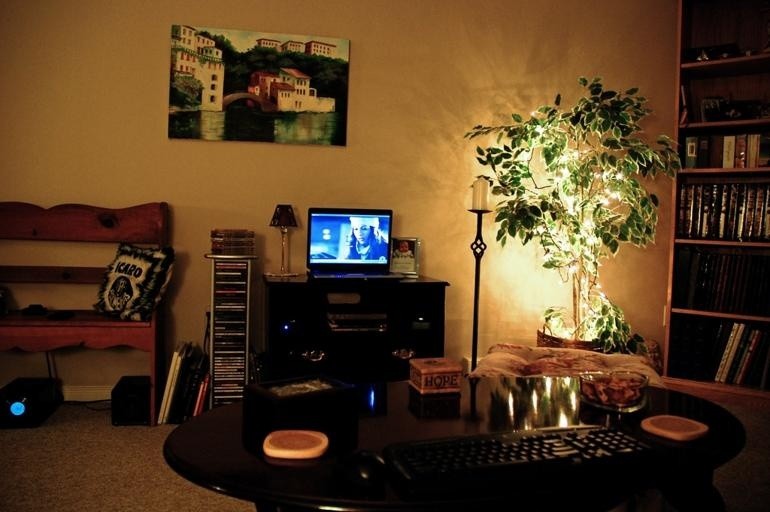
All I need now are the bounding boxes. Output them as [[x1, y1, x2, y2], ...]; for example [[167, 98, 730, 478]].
[[351, 451, 389, 484]]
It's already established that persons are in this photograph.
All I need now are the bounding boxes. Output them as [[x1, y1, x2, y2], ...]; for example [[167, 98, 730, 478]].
[[347, 217, 388, 260], [393, 241, 413, 258]]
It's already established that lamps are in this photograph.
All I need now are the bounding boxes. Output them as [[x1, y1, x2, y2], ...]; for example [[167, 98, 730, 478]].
[[265, 203, 301, 278]]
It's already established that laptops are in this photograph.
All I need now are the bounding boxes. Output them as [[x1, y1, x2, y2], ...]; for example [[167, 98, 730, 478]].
[[306, 207, 405, 278]]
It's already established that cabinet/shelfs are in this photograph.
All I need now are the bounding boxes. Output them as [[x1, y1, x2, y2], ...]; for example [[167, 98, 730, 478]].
[[203, 252, 259, 406], [660, 0, 770, 397], [261, 272, 452, 383]]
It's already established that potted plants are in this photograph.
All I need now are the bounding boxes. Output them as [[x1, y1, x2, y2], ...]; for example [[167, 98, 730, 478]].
[[461, 75, 684, 356]]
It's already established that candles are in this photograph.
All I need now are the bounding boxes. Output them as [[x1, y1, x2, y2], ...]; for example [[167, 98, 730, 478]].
[[470, 177, 491, 210]]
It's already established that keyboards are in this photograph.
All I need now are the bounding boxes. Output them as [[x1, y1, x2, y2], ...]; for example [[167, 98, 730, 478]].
[[384, 432, 650, 486]]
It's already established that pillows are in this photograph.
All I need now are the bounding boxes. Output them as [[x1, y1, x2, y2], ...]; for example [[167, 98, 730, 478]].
[[92, 241, 176, 321]]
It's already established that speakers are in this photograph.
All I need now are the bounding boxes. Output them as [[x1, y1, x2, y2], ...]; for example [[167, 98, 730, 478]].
[[111, 375, 151, 426], [0, 377, 63, 430]]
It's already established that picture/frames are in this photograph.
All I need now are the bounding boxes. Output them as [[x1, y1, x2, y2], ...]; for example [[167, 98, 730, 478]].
[[388, 237, 421, 278]]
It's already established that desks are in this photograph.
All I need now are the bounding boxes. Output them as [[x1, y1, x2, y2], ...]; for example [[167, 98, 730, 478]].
[[162, 379, 750, 512]]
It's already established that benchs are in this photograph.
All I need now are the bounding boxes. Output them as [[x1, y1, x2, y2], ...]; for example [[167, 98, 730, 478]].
[[0, 201, 172, 426]]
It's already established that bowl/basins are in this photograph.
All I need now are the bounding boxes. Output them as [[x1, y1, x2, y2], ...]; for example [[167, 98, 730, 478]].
[[580, 371, 647, 409]]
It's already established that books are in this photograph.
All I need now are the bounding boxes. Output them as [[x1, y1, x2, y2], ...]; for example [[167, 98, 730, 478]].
[[680, 85, 690, 125], [679, 135, 770, 389], [157, 261, 247, 425]]
